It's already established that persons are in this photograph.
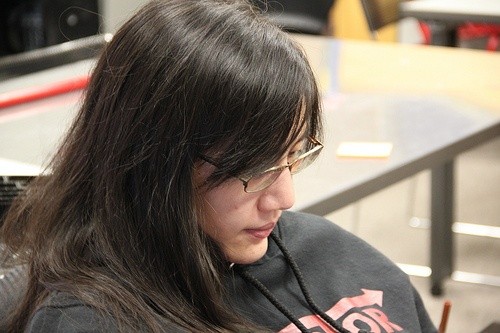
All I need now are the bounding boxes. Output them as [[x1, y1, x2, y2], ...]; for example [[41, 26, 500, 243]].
[[26, 0, 437, 333]]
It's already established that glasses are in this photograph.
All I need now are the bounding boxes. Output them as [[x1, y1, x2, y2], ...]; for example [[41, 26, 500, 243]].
[[198, 135, 325, 193]]
